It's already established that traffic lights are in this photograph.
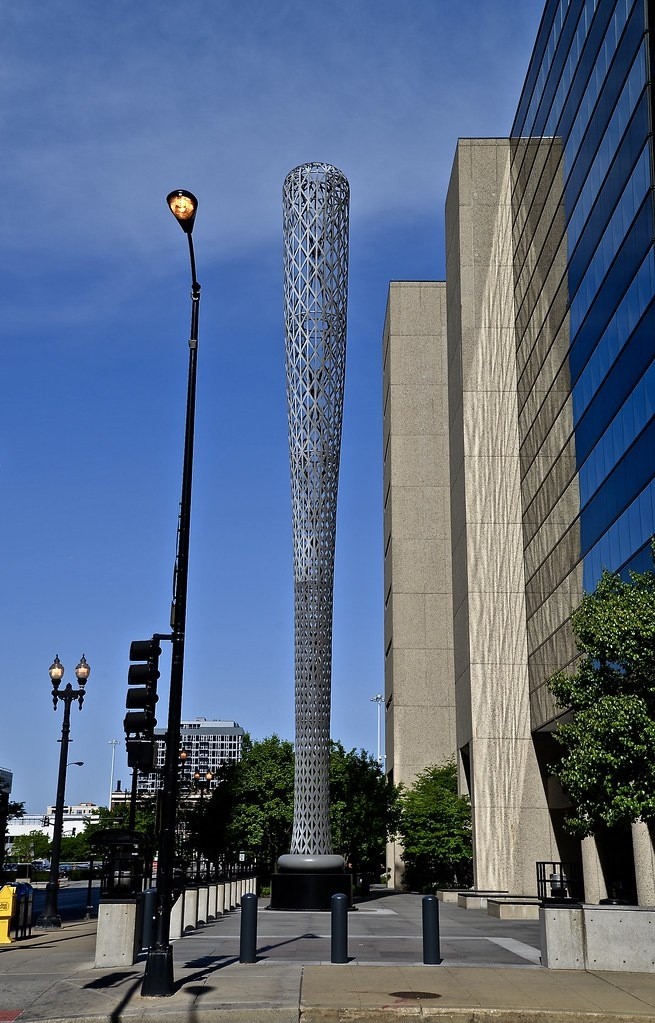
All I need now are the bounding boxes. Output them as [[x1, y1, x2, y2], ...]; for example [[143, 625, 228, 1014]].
[[123, 639, 162, 739]]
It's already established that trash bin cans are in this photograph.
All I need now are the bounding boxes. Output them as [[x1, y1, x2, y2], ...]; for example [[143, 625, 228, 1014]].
[[4, 882, 34, 939]]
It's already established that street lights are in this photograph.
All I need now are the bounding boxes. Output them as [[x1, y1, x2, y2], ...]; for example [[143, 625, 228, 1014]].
[[140, 188, 201, 999], [36, 652, 91, 928], [370, 694, 385, 766]]
